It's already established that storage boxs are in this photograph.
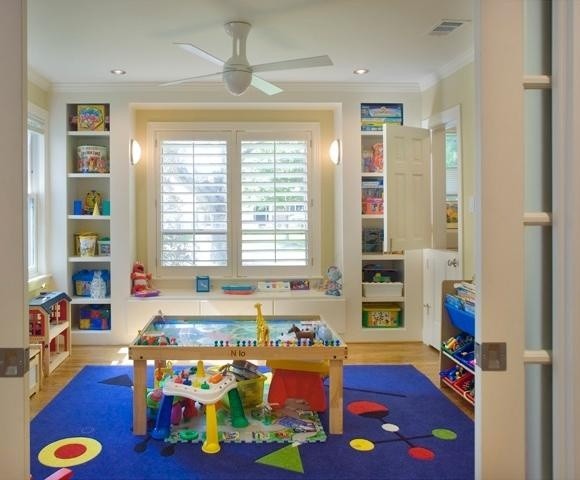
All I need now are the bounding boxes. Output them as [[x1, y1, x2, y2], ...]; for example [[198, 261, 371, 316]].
[[207, 365, 267, 408], [71, 268, 111, 298], [76, 105, 105, 132], [362, 303, 403, 328], [75, 233, 96, 257], [79, 307, 111, 330], [75, 143, 108, 174], [362, 227, 383, 255], [362, 282, 404, 298], [362, 267, 400, 283], [98, 238, 110, 257]]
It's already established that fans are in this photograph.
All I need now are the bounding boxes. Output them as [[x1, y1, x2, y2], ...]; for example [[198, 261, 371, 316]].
[[159, 20, 334, 97]]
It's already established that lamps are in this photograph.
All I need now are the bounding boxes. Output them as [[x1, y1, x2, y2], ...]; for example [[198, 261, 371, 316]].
[[328, 139, 340, 165], [129, 137, 145, 166], [221, 71, 255, 97]]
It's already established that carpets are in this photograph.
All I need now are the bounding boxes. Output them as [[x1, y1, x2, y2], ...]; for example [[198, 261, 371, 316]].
[[30, 363, 474, 480]]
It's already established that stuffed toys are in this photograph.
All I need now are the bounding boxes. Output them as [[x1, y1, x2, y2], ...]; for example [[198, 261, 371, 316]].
[[324, 265, 343, 297], [130, 260, 152, 295]]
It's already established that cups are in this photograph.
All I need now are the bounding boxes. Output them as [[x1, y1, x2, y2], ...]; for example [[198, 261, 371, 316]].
[[73, 200, 82, 215], [102, 199, 110, 215]]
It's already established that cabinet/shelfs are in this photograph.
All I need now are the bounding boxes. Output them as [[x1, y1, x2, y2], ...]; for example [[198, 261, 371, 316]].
[[360, 102, 407, 330], [128, 290, 348, 346], [440, 280, 475, 424], [65, 101, 112, 332], [29, 343, 43, 399], [28, 289, 72, 378]]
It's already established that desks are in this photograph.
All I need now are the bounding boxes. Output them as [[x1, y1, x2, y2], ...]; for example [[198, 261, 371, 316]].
[[128, 314, 349, 436]]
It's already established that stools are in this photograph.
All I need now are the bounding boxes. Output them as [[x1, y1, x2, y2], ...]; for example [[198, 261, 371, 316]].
[[265, 360, 330, 414]]
